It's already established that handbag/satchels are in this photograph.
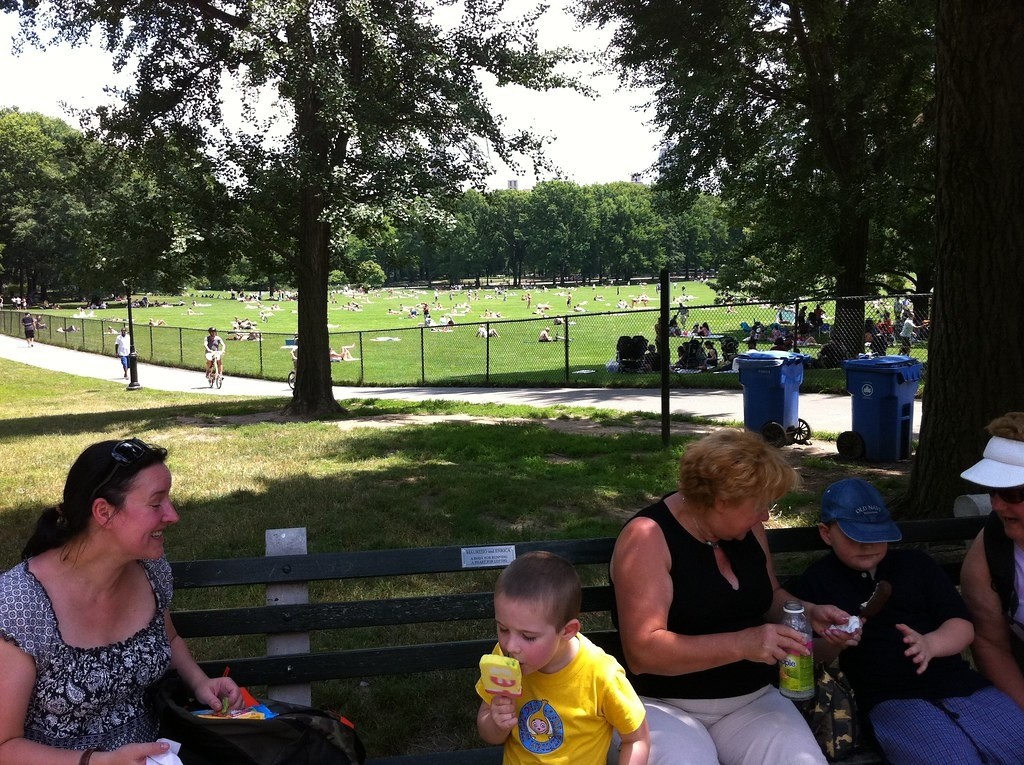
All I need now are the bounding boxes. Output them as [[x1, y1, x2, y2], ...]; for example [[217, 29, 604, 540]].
[[777, 653, 858, 759]]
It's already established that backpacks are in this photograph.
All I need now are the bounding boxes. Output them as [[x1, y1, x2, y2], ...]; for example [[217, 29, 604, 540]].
[[152, 674, 365, 765]]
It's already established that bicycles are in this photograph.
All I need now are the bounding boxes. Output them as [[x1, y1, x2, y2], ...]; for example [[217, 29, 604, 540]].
[[205, 351, 226, 389], [288, 369, 296, 389]]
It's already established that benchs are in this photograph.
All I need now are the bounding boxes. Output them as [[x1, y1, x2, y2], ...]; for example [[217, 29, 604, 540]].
[[152, 519, 989, 765]]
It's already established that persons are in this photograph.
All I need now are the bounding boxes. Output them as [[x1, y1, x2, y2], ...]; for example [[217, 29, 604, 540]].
[[605, 425, 862, 765], [476, 551, 651, 765], [114, 328, 130, 380], [508, 277, 736, 373], [291, 347, 298, 384], [328, 284, 508, 360], [959, 411, 1023, 712], [170, 287, 299, 342], [33, 315, 81, 333], [11, 295, 27, 310], [795, 477, 1024, 765], [720, 289, 929, 358], [0, 296, 4, 311], [203, 327, 226, 379], [44, 292, 170, 334], [0, 439, 245, 765], [21, 313, 36, 348]]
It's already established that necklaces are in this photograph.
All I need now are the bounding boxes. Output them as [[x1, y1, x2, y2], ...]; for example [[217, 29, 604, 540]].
[[681, 495, 720, 549]]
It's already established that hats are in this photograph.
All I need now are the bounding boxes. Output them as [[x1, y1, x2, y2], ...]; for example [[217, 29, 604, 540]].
[[821, 479, 904, 543], [960, 436, 1024, 488]]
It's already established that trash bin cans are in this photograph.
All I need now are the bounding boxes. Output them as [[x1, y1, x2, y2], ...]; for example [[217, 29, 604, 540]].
[[735, 350, 811, 448], [838, 353, 923, 466]]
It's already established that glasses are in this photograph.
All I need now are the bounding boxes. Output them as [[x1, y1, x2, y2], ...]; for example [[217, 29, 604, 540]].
[[986, 487, 1023, 504], [88, 438, 149, 497]]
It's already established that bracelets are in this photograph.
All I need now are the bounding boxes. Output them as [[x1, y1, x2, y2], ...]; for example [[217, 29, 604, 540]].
[[78, 748, 95, 765]]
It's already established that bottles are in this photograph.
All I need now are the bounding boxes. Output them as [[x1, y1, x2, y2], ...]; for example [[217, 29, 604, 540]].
[[780, 600, 816, 702]]
